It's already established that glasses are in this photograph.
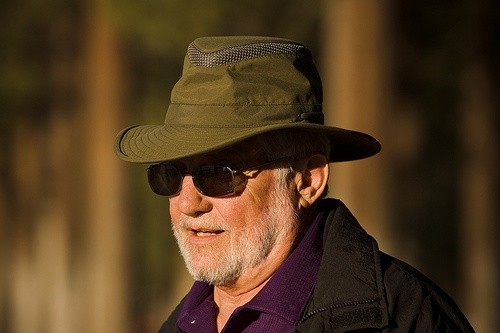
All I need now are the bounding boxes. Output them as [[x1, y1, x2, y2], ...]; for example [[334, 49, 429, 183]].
[[146, 152, 310, 197]]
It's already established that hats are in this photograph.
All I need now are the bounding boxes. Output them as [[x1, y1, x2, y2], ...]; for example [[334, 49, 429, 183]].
[[113, 35, 382, 163]]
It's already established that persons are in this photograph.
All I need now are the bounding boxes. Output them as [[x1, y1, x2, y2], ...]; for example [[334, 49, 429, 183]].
[[112, 36, 474, 333]]
